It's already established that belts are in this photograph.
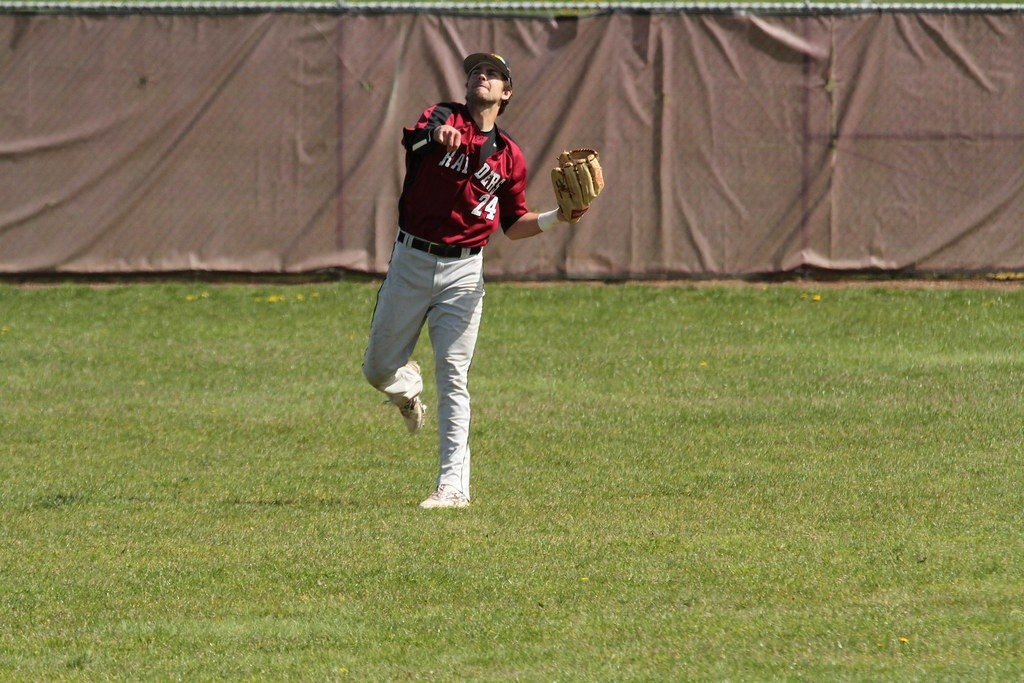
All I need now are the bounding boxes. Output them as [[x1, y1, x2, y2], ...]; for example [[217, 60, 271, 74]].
[[398, 231, 481, 258]]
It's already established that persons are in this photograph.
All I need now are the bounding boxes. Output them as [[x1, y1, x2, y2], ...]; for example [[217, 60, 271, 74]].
[[361, 52, 604, 508]]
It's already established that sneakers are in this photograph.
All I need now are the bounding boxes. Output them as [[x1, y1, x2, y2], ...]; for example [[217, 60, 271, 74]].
[[419, 484, 469, 509], [397, 361, 426, 432]]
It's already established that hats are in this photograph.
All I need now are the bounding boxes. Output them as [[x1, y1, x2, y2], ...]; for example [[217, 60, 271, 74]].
[[463, 52, 512, 84]]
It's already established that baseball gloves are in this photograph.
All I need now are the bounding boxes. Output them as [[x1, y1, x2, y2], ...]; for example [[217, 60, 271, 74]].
[[552, 147, 605, 222]]
